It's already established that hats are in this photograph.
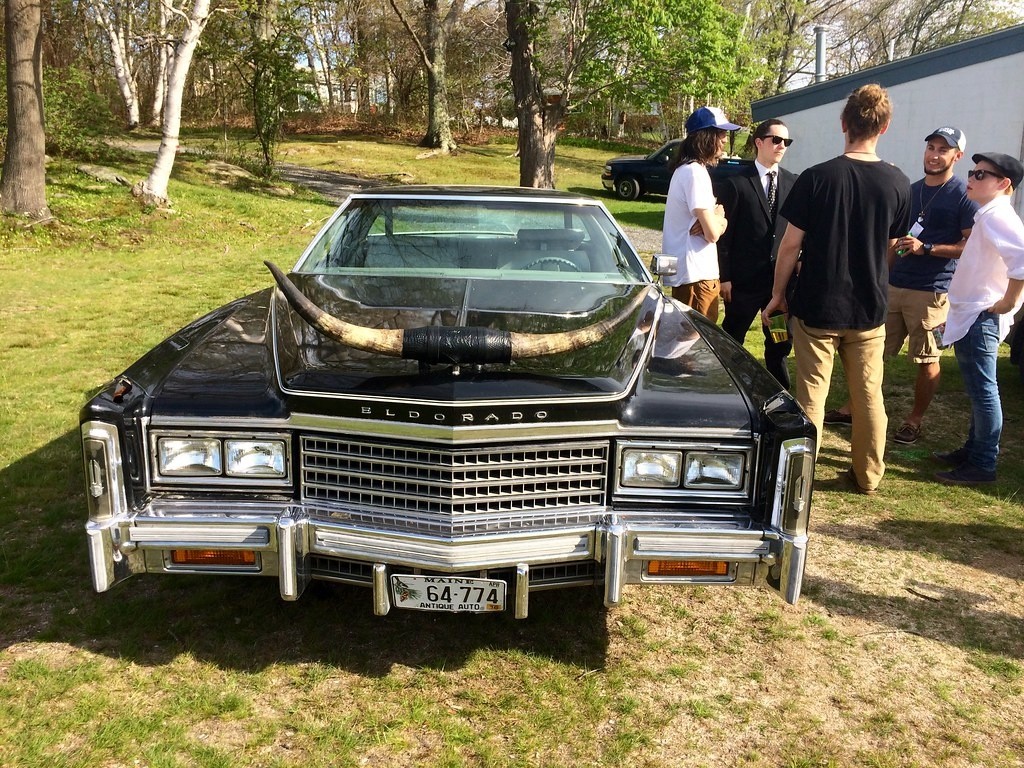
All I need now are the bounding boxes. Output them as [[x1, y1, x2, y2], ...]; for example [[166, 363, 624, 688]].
[[687, 106, 741, 131], [971, 151, 1024, 191], [925, 126, 966, 153]]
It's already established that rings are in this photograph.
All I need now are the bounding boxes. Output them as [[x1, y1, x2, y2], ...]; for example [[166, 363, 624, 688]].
[[899, 240, 903, 243], [899, 245, 902, 248]]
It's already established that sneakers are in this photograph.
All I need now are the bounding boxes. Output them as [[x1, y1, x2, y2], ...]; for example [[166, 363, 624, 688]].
[[931, 451, 959, 467], [935, 464, 996, 485]]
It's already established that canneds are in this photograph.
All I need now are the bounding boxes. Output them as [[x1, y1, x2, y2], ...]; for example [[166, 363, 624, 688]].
[[896, 232, 913, 256]]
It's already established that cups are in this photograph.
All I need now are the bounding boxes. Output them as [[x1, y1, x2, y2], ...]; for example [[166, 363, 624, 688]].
[[767, 314, 788, 343]]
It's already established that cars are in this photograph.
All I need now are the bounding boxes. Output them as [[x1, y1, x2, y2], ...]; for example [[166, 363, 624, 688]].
[[601, 140, 756, 201], [78, 186, 820, 619]]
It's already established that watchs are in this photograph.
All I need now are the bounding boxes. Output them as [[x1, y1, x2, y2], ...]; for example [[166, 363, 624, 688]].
[[922, 242, 934, 255]]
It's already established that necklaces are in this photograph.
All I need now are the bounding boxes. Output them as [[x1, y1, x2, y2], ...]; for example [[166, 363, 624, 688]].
[[844, 151, 876, 155], [918, 176, 952, 222]]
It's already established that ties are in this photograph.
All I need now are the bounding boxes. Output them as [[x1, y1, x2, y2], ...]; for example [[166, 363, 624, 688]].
[[765, 171, 778, 225]]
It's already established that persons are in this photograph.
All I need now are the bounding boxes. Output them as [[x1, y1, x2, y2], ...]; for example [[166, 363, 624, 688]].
[[661, 105, 742, 324], [759, 82, 913, 495], [628, 283, 710, 377], [715, 117, 803, 390], [936, 150, 1024, 482], [823, 126, 981, 445]]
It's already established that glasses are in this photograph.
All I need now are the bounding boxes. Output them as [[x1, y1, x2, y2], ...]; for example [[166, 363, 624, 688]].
[[968, 170, 1004, 182], [760, 135, 793, 146]]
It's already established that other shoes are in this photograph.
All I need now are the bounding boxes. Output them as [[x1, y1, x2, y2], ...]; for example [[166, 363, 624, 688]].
[[824, 409, 852, 426], [893, 423, 921, 445], [848, 464, 877, 494]]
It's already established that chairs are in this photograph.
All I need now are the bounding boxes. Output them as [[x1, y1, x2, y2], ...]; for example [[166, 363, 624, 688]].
[[495, 228, 591, 272]]
